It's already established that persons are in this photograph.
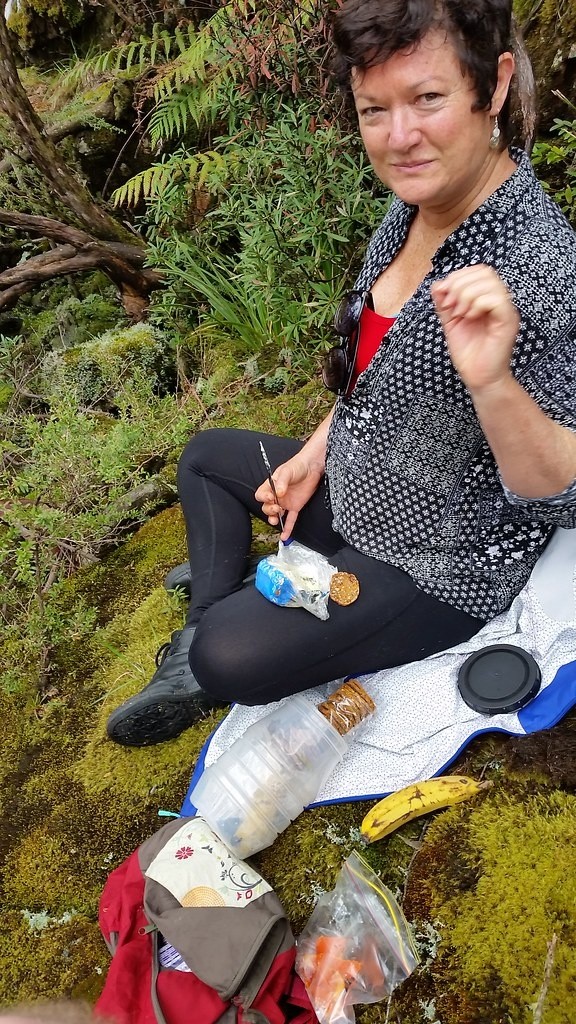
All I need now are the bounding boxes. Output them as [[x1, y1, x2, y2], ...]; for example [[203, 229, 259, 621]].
[[105, 0, 576, 747]]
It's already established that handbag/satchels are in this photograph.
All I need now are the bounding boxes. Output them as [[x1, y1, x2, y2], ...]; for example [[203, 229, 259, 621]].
[[94, 815, 318, 1023]]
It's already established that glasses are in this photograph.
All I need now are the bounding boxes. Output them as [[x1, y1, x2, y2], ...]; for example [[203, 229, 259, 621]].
[[322, 289, 374, 396]]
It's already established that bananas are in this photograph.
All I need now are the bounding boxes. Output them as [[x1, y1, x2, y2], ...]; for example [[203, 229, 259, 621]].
[[360, 775, 495, 845], [231, 773, 286, 844]]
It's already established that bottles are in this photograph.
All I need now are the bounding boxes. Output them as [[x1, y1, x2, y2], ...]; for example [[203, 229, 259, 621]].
[[190, 678, 375, 861]]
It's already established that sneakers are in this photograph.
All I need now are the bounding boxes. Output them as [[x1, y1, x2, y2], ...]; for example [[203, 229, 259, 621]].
[[164, 555, 271, 600], [106, 628, 231, 746]]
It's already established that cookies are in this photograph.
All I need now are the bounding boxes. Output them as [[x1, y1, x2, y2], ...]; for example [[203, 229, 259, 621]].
[[272, 680, 377, 772], [328, 572, 359, 606]]
